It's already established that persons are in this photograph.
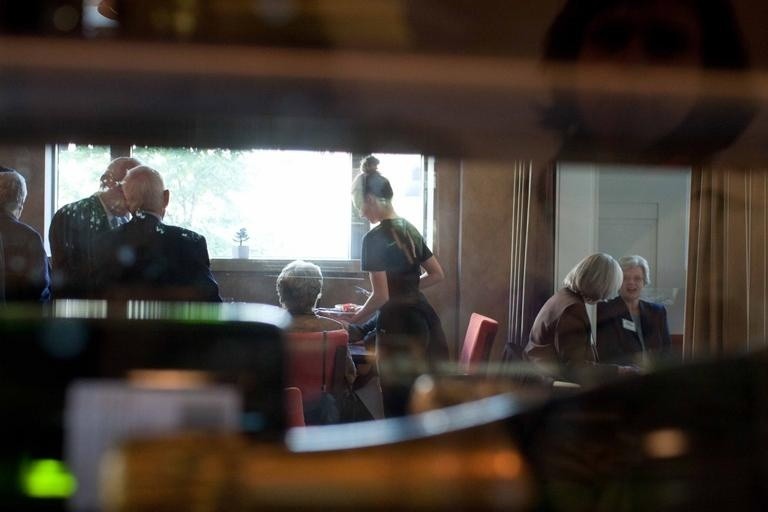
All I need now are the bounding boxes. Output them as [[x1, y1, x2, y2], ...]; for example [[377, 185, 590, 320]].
[[524, 252, 642, 386], [48, 156, 144, 300], [0, 167, 55, 303], [272, 258, 358, 428], [340, 155, 452, 419], [596, 253, 672, 376], [83, 164, 225, 304]]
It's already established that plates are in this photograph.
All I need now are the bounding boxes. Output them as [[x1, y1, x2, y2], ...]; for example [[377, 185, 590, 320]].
[[317, 306, 360, 318]]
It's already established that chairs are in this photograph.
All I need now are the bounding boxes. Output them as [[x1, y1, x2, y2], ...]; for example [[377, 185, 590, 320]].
[[280, 326, 350, 428], [432, 312, 582, 400]]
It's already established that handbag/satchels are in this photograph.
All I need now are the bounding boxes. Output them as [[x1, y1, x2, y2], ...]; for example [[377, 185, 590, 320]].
[[317, 387, 374, 426]]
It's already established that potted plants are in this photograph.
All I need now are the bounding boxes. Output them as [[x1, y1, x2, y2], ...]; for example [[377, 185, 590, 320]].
[[231, 227, 250, 259]]
